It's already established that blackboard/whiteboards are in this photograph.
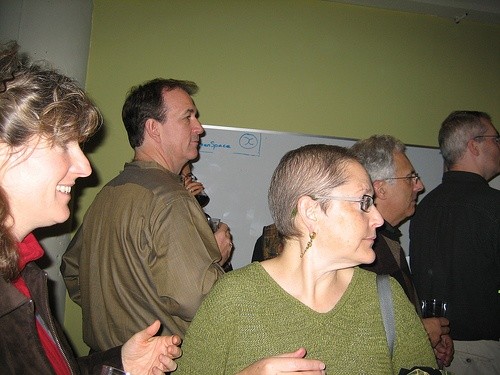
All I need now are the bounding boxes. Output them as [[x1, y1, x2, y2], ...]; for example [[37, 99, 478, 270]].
[[192, 125, 500, 270]]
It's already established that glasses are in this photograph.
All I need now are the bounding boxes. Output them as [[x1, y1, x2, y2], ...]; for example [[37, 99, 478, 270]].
[[384, 173, 418, 184], [179, 172, 197, 186], [290, 194, 373, 216]]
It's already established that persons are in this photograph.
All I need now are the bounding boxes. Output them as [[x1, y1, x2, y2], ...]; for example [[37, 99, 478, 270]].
[[0, 40, 182, 375], [179, 162, 204, 196], [250, 223, 284, 262], [348, 134, 456, 368], [409, 111, 500, 375], [167, 144, 451, 375], [60, 78, 232, 356]]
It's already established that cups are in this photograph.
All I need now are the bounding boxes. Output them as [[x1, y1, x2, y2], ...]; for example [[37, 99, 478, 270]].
[[419, 296, 447, 317], [207, 218, 221, 234]]
